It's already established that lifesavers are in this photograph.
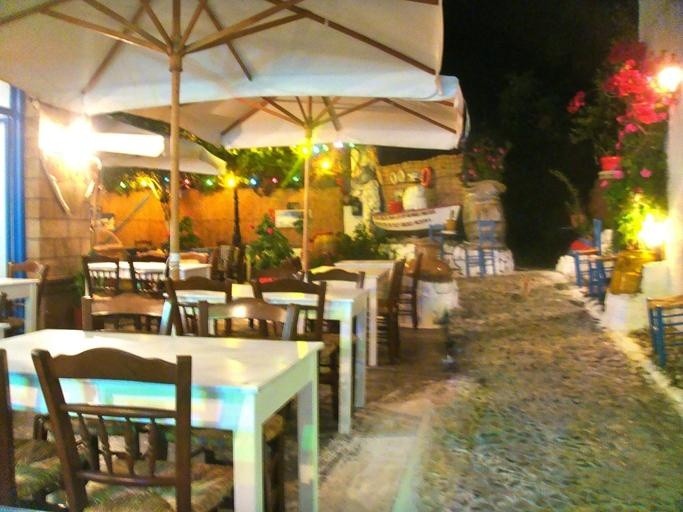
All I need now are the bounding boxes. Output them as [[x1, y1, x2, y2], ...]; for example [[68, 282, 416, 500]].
[[419, 168, 432, 188]]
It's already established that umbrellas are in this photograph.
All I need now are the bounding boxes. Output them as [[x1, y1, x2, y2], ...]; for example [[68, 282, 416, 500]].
[[0, 0, 445, 291], [118, 74, 472, 282], [94, 116, 227, 178]]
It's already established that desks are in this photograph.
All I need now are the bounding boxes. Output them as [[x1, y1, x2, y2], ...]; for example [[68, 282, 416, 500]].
[[1, 327, 326, 512]]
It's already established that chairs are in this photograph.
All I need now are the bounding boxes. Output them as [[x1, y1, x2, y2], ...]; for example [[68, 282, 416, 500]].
[[566, 218, 683, 367], [34, 295, 174, 463], [28, 348, 236, 512], [3, 352, 90, 511], [427, 217, 510, 278], [158, 300, 300, 512], [0, 240, 426, 435]]
